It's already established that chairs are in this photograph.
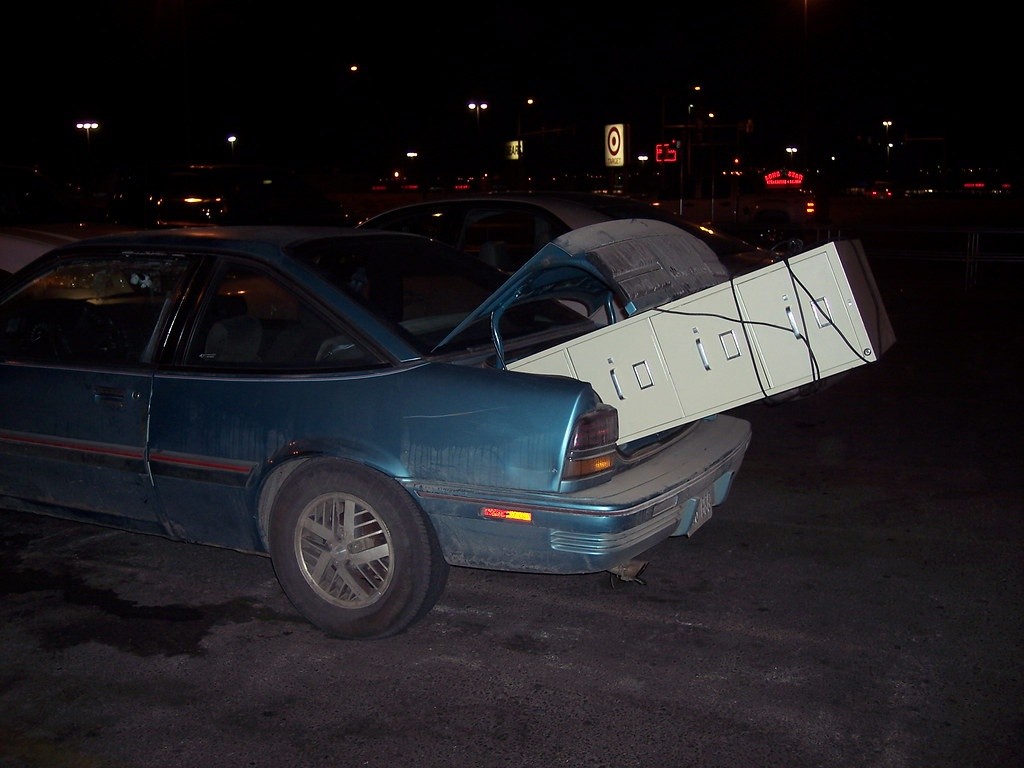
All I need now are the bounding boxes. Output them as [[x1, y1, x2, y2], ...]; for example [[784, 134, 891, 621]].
[[204, 313, 264, 364], [271, 293, 341, 364]]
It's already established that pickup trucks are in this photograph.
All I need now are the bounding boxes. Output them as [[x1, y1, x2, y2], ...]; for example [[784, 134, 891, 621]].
[[645, 164, 817, 249]]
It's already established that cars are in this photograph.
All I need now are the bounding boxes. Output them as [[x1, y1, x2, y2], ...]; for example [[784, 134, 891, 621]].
[[362, 193, 792, 277], [0, 218, 752, 641], [1, 224, 178, 355]]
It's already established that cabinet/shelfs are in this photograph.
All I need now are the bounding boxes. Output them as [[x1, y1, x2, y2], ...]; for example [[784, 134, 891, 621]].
[[503, 231, 891, 450]]
